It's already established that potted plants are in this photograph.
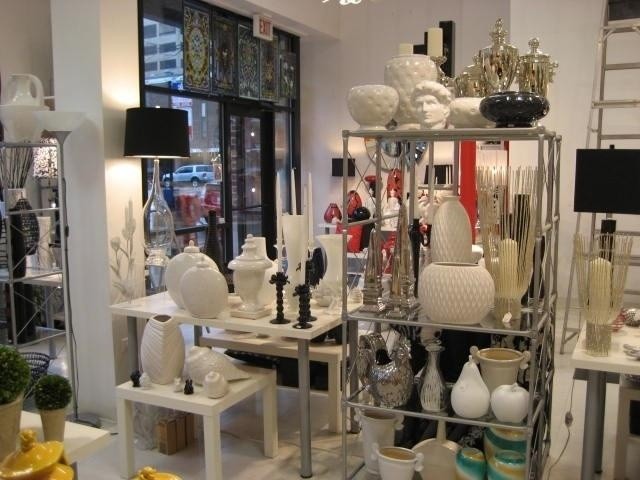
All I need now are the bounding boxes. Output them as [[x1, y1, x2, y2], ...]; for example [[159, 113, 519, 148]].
[[0, 343, 73, 459]]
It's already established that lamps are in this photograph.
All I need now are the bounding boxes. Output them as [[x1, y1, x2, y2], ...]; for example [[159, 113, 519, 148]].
[[122, 104, 191, 265], [34, 109, 102, 428], [573, 144, 640, 309]]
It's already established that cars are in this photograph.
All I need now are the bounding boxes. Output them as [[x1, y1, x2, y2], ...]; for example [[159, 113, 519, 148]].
[[161, 164, 215, 188]]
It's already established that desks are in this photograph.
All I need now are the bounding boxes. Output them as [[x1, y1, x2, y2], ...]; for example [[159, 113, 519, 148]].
[[20, 409, 109, 480], [572, 319, 640, 480], [109, 286, 364, 478]]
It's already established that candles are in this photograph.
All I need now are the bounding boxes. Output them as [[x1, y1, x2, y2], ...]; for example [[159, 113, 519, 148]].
[[427, 28, 444, 57], [398, 42, 414, 54], [275, 167, 315, 287]]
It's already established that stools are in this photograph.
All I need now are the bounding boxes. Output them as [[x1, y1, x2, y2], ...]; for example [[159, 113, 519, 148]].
[[116, 366, 279, 480], [200, 327, 350, 435]]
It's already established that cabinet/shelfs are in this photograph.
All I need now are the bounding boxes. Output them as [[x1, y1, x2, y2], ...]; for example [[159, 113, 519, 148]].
[[339, 129, 563, 480], [0, 140, 67, 361]]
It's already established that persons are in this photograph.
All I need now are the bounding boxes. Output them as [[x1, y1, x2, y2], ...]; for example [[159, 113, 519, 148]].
[[411, 80, 452, 130]]
